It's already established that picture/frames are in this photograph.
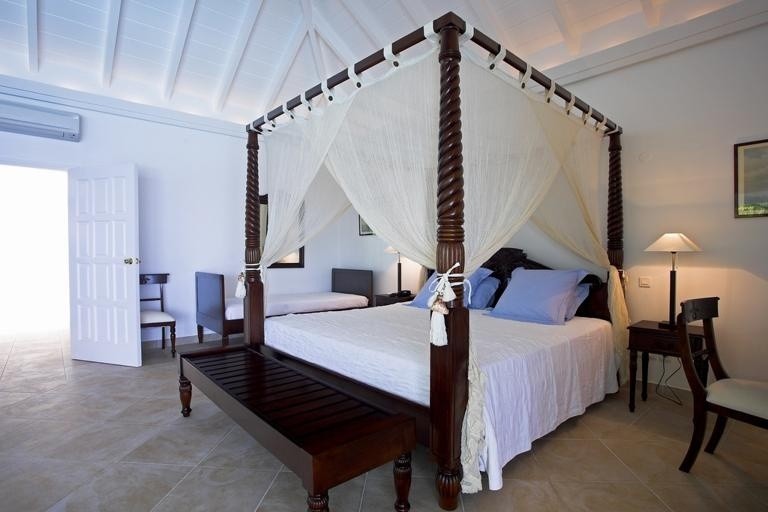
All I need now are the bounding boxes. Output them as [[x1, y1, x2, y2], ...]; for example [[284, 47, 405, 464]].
[[734, 139, 768, 218], [359, 214, 374, 235]]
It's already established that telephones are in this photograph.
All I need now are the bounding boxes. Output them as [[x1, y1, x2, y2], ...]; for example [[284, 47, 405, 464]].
[[397, 290, 411, 297]]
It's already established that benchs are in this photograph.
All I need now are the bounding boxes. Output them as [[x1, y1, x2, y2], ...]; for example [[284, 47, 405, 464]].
[[179, 345, 417, 512]]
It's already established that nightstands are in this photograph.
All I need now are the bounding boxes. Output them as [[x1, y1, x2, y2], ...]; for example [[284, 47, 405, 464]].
[[628, 321, 709, 413], [374, 294, 416, 306]]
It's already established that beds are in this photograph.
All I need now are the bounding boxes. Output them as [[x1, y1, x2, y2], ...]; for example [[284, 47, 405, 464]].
[[194, 268, 373, 343], [242, 12, 623, 495]]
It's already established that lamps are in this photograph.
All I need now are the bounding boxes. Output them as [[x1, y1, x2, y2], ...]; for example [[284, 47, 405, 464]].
[[643, 232, 703, 329], [385, 245, 401, 292]]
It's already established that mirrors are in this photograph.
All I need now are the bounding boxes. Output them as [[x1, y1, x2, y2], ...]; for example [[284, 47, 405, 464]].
[[259, 191, 304, 268]]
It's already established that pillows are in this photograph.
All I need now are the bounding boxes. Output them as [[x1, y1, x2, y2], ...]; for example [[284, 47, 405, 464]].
[[469, 276, 500, 310], [405, 267, 496, 309], [483, 267, 590, 324], [507, 276, 591, 320]]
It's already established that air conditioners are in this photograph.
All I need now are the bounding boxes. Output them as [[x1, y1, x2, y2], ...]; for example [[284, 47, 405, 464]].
[[0, 100, 80, 142]]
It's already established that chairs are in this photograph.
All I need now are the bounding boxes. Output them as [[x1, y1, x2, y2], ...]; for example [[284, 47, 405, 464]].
[[140, 274, 176, 358], [677, 296, 767, 473]]
